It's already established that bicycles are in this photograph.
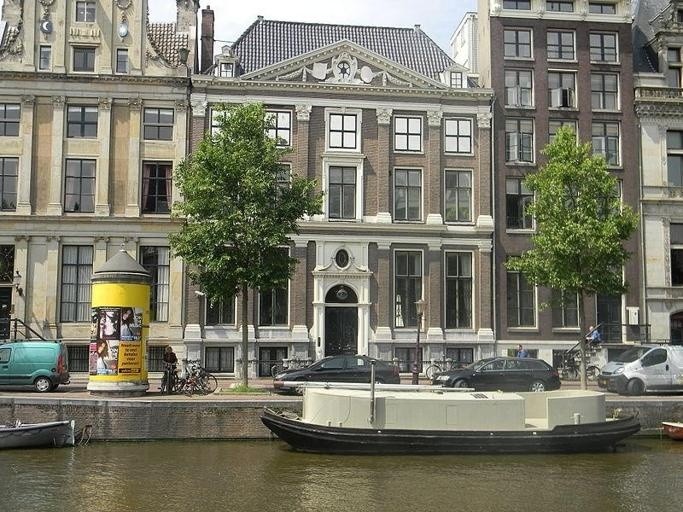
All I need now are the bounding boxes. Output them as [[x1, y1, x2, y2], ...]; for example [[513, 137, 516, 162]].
[[163, 359, 217, 396], [271, 356, 304, 377], [566, 360, 600, 381], [426, 359, 464, 379]]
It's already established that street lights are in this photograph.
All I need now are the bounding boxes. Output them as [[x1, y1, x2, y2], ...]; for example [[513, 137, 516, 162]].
[[411, 299, 427, 385]]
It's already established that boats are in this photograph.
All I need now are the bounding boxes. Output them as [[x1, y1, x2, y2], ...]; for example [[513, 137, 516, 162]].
[[260, 388, 641, 457], [0, 418, 75, 448]]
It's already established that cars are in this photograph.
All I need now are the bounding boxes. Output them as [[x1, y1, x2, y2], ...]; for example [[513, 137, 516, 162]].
[[273, 354, 399, 396], [431, 357, 560, 394]]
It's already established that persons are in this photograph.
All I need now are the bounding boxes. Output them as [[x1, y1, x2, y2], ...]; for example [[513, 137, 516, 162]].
[[516, 343, 528, 358], [120, 307, 135, 341], [89, 307, 118, 338], [94, 340, 107, 375], [585, 326, 601, 343], [160, 347, 177, 388]]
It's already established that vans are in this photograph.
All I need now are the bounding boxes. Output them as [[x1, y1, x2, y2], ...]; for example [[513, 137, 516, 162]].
[[597, 345, 682, 396], [1, 341, 70, 392]]
